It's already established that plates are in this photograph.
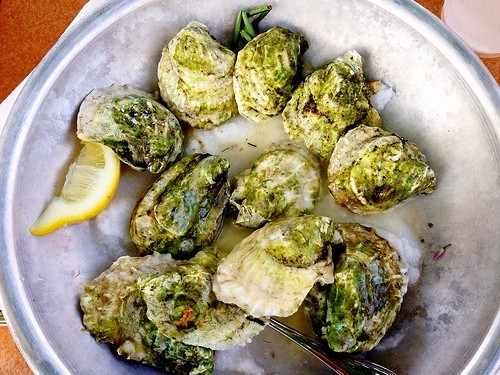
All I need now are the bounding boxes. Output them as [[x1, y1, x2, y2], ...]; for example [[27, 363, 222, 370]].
[[0, 0, 500, 375]]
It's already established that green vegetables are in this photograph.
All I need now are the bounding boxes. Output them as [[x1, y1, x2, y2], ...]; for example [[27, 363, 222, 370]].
[[232, 4, 272, 53]]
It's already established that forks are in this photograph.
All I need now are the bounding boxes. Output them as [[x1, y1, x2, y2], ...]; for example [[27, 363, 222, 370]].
[[266, 317, 395, 375]]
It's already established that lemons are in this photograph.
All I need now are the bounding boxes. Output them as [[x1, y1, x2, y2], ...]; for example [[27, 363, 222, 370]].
[[30, 144, 120, 237]]
